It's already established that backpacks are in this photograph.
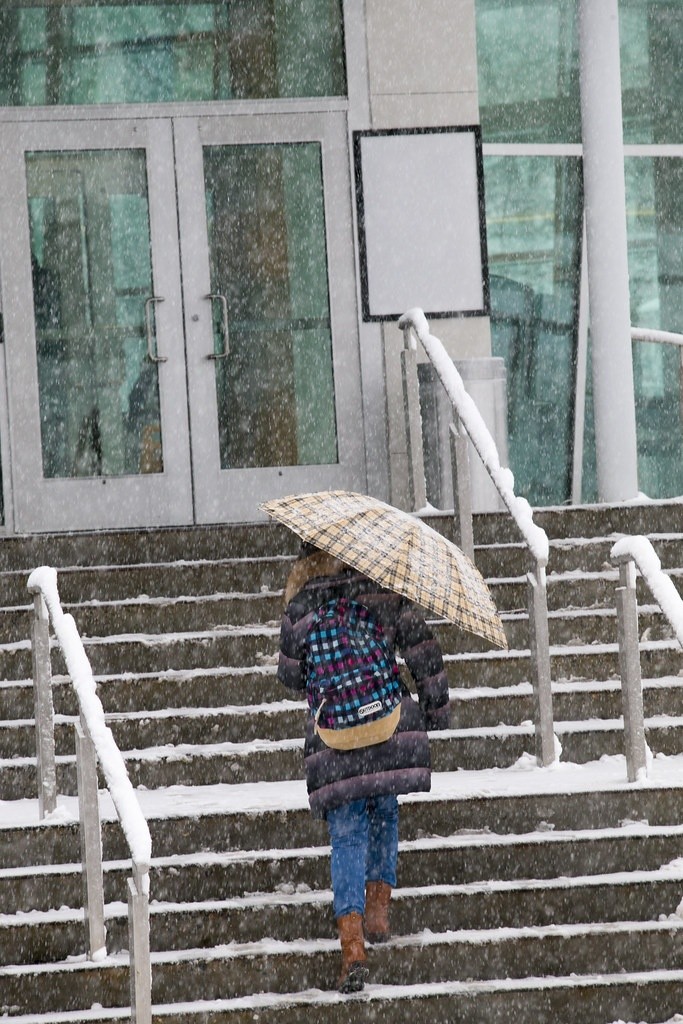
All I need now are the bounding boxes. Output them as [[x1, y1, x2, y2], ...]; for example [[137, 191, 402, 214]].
[[305, 580, 404, 751]]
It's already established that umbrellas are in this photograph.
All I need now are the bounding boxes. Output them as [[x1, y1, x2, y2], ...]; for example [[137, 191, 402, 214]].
[[258, 490, 509, 650]]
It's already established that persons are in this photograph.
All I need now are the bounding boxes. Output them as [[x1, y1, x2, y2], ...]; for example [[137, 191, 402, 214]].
[[280, 542, 453, 993]]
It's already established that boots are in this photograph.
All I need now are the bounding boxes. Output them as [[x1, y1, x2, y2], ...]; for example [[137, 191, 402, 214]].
[[337, 911, 366, 994], [365, 881, 392, 943]]
